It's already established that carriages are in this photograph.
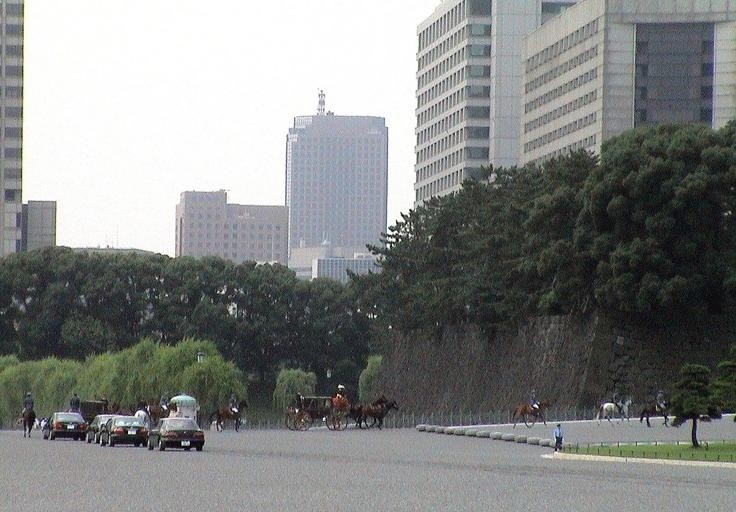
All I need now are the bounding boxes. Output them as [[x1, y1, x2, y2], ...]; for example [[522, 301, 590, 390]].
[[285, 392, 399, 431]]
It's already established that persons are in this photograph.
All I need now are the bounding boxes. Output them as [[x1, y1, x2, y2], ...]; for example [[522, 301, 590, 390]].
[[22, 392, 34, 417], [530, 390, 539, 417], [138, 399, 150, 417], [656, 391, 666, 409], [553, 423, 563, 451], [70, 393, 79, 412], [336, 384, 345, 397], [296, 390, 303, 410], [161, 392, 169, 418], [230, 393, 239, 416], [613, 388, 621, 411], [100, 393, 108, 409]]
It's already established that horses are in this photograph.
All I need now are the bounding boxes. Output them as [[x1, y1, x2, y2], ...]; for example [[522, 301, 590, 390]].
[[355, 394, 399, 430], [22, 409, 36, 438], [640, 402, 672, 428], [145, 401, 178, 423], [208, 399, 248, 433], [512, 395, 552, 429], [595, 395, 635, 427]]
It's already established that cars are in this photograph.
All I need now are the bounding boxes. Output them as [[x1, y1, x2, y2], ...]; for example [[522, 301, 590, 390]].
[[32, 394, 205, 451]]
